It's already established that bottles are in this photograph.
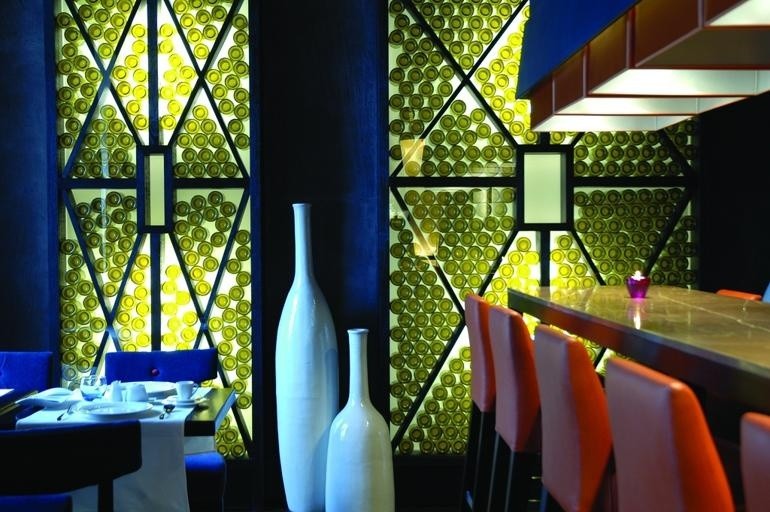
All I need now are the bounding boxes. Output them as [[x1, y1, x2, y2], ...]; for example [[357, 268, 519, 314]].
[[126, 384, 148, 402]]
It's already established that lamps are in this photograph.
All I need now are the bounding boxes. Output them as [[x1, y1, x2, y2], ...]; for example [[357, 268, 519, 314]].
[[528, 2, 769, 135]]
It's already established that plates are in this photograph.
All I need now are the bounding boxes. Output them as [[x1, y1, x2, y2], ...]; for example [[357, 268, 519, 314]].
[[78, 401, 155, 417], [162, 395, 207, 407], [127, 380, 176, 394]]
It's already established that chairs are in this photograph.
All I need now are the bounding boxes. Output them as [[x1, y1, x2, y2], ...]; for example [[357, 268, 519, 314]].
[[458, 284, 769, 512], [0, 347, 235, 511]]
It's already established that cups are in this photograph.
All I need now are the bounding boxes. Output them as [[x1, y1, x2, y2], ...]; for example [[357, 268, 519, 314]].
[[623, 274, 650, 297], [175, 381, 198, 399], [109, 381, 124, 403], [78, 376, 109, 404]]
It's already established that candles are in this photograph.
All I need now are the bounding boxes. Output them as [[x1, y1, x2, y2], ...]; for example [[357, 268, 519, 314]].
[[626, 271, 650, 298]]
